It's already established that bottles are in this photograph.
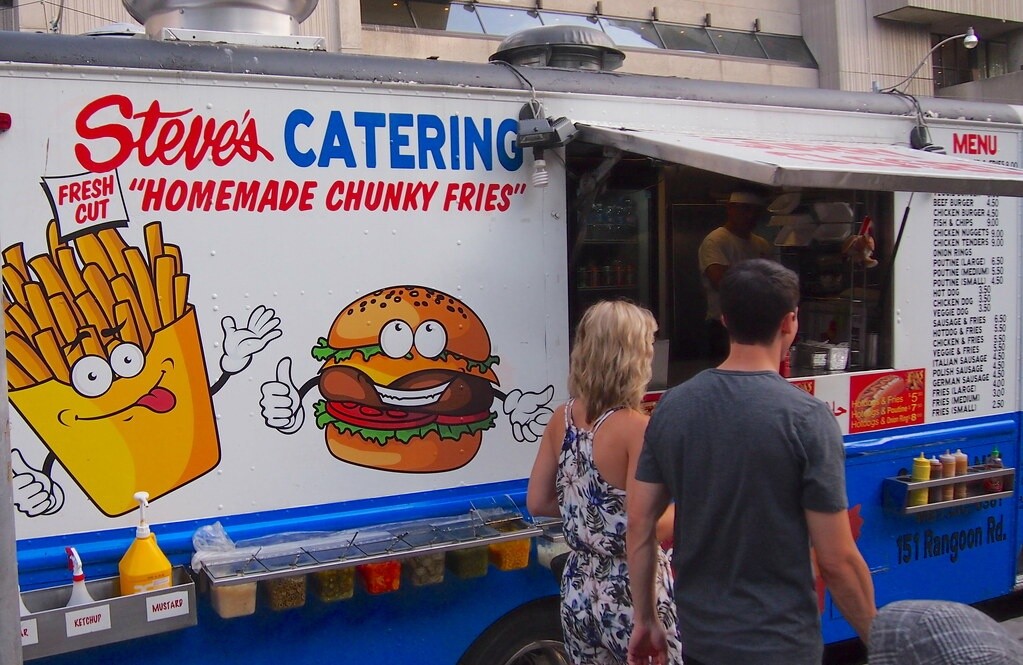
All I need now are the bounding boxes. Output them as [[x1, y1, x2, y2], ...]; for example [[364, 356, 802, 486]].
[[928, 455, 943, 504], [951, 449, 968, 499], [939, 449, 956, 500], [909, 452, 931, 506], [983, 446, 1005, 493]]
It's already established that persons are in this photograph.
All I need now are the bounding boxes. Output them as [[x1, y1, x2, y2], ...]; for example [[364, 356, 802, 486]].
[[527, 299, 684, 665], [697, 193, 769, 364], [869, 599, 1023, 665], [627, 258, 877, 665]]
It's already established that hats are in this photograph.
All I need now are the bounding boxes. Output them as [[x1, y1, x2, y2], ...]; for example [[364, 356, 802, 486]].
[[729, 192, 761, 204]]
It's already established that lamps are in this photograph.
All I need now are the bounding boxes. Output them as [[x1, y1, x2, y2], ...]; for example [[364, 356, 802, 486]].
[[596, 1, 602, 15], [873, 27, 979, 92], [536, 1, 543, 9], [706, 13, 712, 27], [755, 18, 761, 32], [654, 7, 659, 20]]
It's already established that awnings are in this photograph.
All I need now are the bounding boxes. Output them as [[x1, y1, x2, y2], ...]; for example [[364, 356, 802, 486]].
[[572, 118, 1023, 311]]
